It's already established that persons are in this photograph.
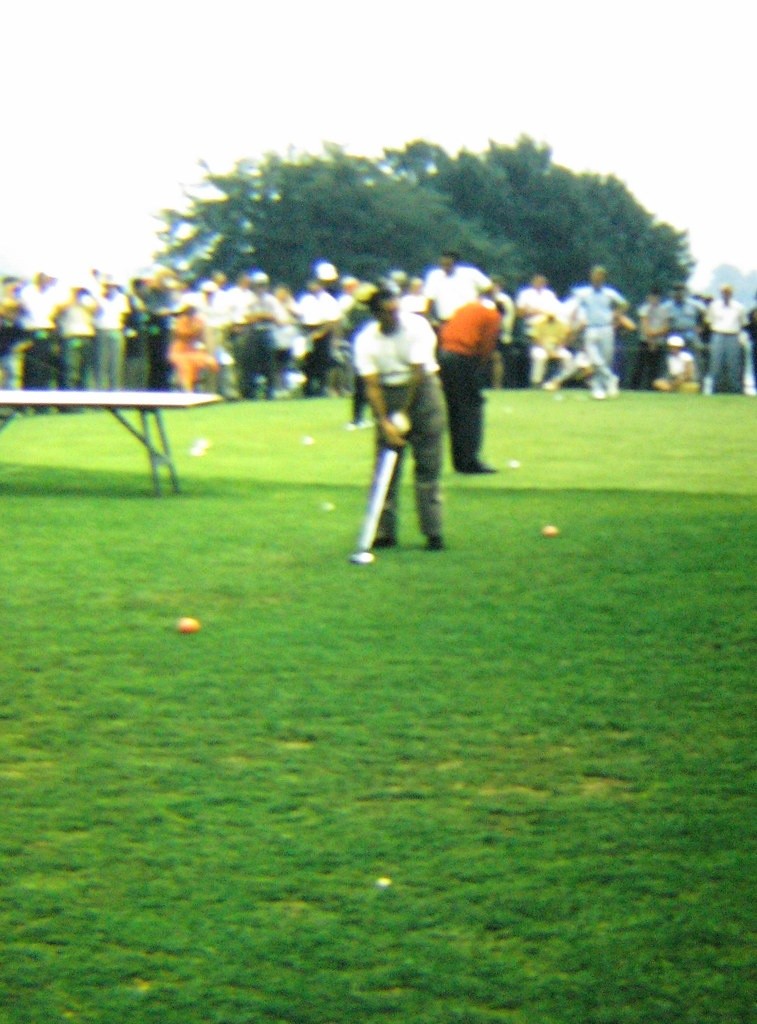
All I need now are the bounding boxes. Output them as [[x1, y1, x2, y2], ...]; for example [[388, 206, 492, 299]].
[[437, 300, 507, 473], [353, 290, 446, 552], [0, 251, 757, 402]]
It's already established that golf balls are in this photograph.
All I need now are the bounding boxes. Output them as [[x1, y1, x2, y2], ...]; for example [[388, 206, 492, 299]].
[[188, 437, 211, 457], [177, 617, 200, 634], [303, 436, 314, 445], [358, 552, 375, 564], [543, 525, 558, 536], [324, 503, 333, 511], [508, 459, 520, 468]]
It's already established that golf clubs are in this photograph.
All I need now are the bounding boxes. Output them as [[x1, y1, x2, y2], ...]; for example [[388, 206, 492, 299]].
[[351, 446, 399, 564]]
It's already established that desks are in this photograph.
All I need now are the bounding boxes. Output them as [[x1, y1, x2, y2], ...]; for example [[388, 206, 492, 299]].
[[0, 390, 224, 498]]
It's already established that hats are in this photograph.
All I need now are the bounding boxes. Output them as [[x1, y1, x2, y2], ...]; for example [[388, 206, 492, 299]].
[[252, 273, 269, 285], [316, 263, 338, 282], [668, 336, 686, 348]]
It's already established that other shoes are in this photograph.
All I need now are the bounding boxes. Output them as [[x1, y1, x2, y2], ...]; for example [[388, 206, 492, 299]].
[[426, 538, 444, 551], [475, 465, 496, 473], [373, 537, 396, 549]]
